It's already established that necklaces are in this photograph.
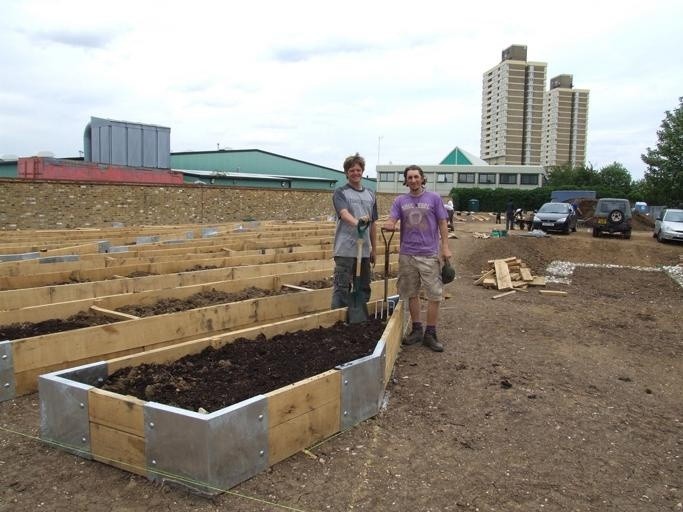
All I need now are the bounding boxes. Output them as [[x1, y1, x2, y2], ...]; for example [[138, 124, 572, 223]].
[[410, 196, 421, 208]]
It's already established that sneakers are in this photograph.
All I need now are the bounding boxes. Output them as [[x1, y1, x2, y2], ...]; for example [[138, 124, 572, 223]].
[[405, 327, 423, 345], [423, 332, 443, 351]]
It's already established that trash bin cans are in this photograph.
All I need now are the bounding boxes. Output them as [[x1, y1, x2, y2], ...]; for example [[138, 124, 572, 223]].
[[469, 199, 480, 212]]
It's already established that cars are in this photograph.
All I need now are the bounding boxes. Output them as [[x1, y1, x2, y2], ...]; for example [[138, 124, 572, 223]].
[[654, 207, 683, 244], [532, 202, 577, 235], [635, 201, 648, 213]]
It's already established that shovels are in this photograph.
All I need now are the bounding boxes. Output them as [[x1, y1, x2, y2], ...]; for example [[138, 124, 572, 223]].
[[347, 218, 370, 323]]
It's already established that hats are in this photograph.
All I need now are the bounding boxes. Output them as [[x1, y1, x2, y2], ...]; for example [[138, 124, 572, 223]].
[[441, 258, 455, 283]]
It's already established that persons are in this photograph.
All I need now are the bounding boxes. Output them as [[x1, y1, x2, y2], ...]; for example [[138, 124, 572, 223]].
[[331, 152, 378, 312], [572, 198, 582, 232], [513, 207, 524, 218], [503, 199, 516, 230], [384, 164, 453, 353], [495, 210, 501, 224]]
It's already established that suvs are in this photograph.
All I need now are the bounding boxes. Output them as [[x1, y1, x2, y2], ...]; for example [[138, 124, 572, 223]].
[[592, 197, 632, 238]]
